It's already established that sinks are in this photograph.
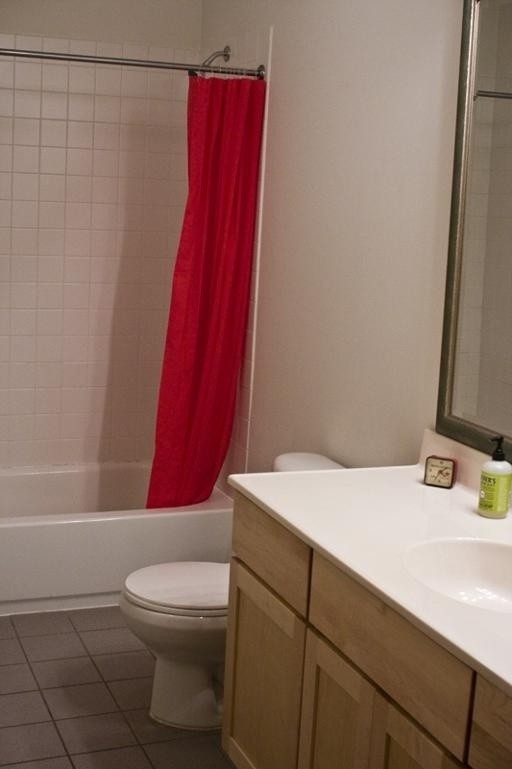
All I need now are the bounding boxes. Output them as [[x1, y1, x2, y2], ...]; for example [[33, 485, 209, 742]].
[[401, 537, 512, 614]]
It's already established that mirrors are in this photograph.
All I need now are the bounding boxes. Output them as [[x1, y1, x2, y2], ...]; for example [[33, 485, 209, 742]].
[[434, 3, 512, 468]]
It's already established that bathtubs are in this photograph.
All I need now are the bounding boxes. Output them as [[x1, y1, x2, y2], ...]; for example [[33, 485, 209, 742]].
[[0, 454, 237, 616]]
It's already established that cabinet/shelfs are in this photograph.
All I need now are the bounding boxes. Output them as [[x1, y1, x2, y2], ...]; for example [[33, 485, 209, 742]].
[[221, 461, 512, 769]]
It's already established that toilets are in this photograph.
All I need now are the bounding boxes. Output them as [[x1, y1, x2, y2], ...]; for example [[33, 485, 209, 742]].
[[116, 450, 349, 732]]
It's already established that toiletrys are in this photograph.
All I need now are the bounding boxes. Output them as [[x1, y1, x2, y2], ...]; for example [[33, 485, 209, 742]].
[[476, 434, 512, 519]]
